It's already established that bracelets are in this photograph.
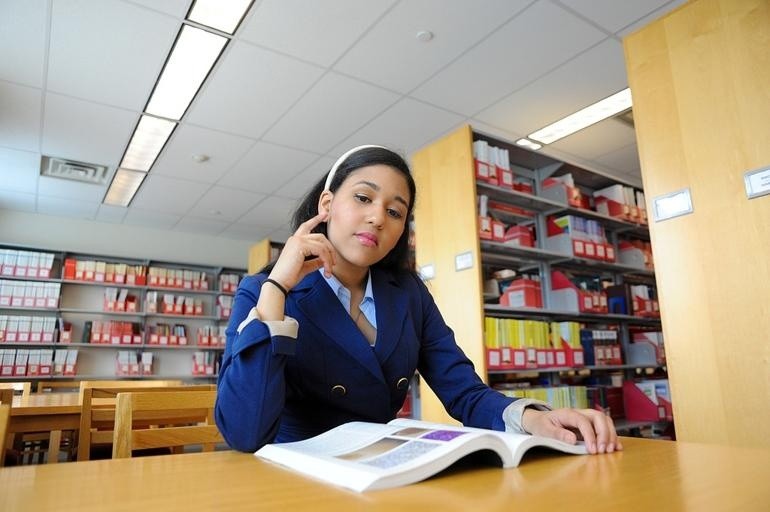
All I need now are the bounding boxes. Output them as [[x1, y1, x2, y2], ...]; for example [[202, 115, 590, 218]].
[[261, 279, 288, 299]]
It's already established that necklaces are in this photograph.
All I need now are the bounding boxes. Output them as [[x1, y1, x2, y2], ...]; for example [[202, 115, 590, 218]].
[[356, 311, 362, 323]]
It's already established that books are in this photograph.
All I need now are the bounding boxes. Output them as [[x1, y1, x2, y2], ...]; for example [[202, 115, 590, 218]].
[[488, 369, 623, 421], [474, 140, 510, 170], [477, 195, 488, 217], [483, 269, 660, 318], [631, 240, 654, 269], [1, 248, 244, 374], [485, 316, 623, 366], [553, 173, 646, 209], [554, 215, 607, 244], [629, 326, 670, 406], [255, 417, 593, 493]]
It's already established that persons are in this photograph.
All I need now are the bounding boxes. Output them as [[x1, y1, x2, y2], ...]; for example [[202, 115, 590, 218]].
[[215, 142, 624, 455]]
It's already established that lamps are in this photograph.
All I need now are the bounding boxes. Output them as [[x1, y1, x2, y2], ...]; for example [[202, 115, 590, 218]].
[[515, 85, 634, 152], [100, 0, 255, 207]]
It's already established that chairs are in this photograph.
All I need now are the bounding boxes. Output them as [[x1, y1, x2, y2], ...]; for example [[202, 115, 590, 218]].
[[0, 380, 225, 467]]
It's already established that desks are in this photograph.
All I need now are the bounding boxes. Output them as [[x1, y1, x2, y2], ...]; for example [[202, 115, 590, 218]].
[[1, 435, 769, 512]]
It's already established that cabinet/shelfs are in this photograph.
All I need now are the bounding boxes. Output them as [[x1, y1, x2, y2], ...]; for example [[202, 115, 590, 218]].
[[0, 239, 287, 382], [394, 0, 770, 441]]
